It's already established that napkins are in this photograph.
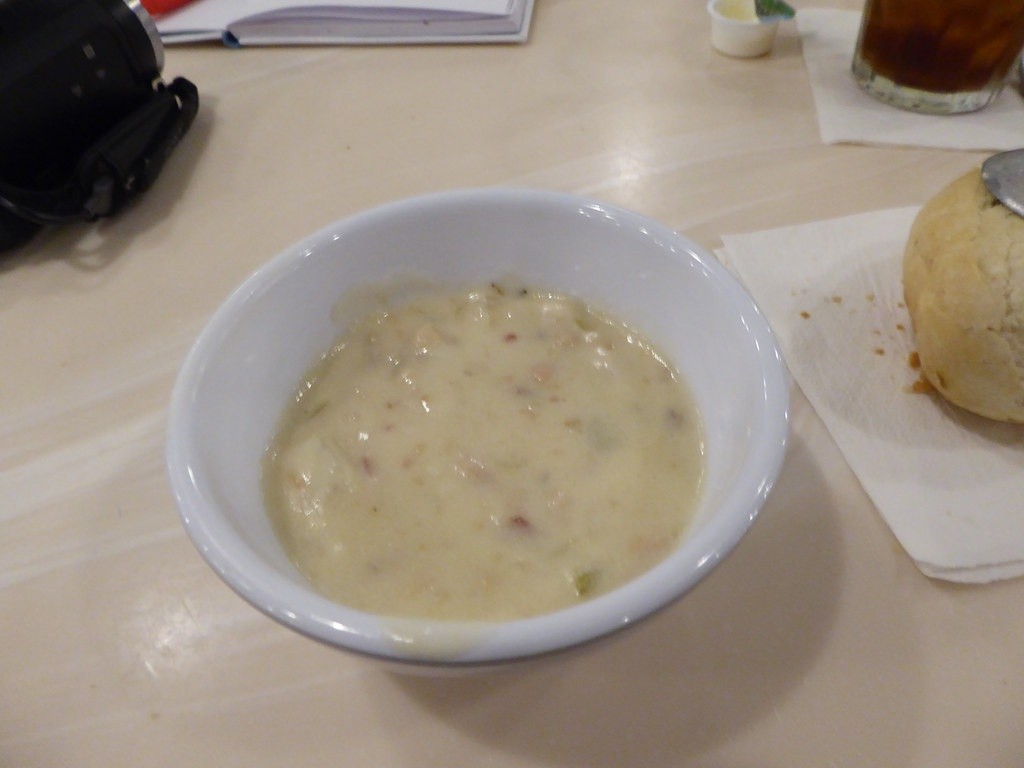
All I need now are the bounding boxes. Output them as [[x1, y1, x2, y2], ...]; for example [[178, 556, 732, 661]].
[[719, 205, 1024, 585], [796, 7, 1024, 151]]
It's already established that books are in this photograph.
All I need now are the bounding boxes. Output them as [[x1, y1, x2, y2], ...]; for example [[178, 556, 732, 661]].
[[146, 1, 537, 50]]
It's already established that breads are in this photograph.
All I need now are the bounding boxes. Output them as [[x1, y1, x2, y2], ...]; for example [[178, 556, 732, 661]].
[[904, 167, 1024, 426]]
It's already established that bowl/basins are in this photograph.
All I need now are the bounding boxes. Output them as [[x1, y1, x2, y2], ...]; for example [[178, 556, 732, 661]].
[[165, 188, 790, 663]]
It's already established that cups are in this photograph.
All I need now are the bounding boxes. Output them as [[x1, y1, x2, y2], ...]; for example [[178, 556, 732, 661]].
[[851, 0, 1024, 115], [707, 0, 779, 57]]
